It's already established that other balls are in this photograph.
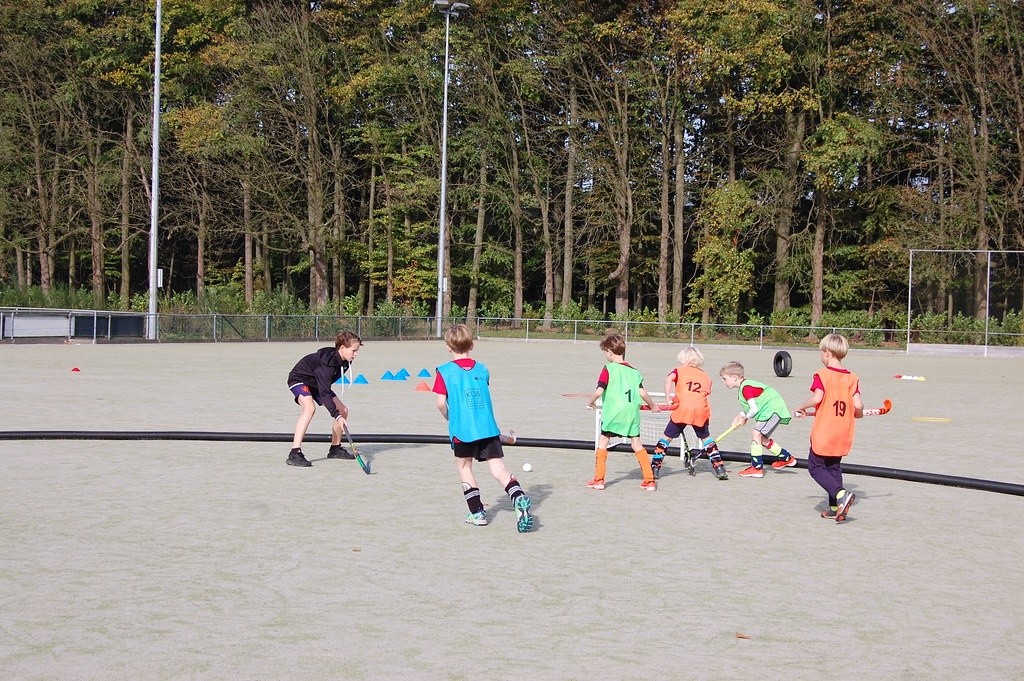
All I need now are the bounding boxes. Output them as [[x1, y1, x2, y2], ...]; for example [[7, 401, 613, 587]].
[[522, 463, 532, 472]]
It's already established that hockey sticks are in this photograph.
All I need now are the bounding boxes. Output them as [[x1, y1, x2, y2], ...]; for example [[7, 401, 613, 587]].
[[685, 421, 740, 467], [586, 393, 680, 411], [795, 399, 892, 418], [681, 430, 695, 475], [499, 430, 517, 444], [343, 424, 371, 475]]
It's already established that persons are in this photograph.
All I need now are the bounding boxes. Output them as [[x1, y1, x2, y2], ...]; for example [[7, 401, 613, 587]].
[[718, 360, 796, 477], [286, 330, 362, 467], [589, 333, 661, 490], [798, 333, 863, 521], [650, 348, 727, 478], [432, 324, 534, 534]]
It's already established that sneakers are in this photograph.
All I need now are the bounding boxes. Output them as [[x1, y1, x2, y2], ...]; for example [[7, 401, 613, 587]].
[[821, 507, 847, 520], [653, 467, 659, 479], [512, 493, 533, 533], [286, 448, 311, 467], [738, 466, 764, 478], [640, 480, 656, 491], [715, 466, 728, 480], [586, 477, 605, 490], [465, 510, 488, 526], [327, 444, 355, 459], [772, 455, 797, 470], [835, 491, 855, 521]]
